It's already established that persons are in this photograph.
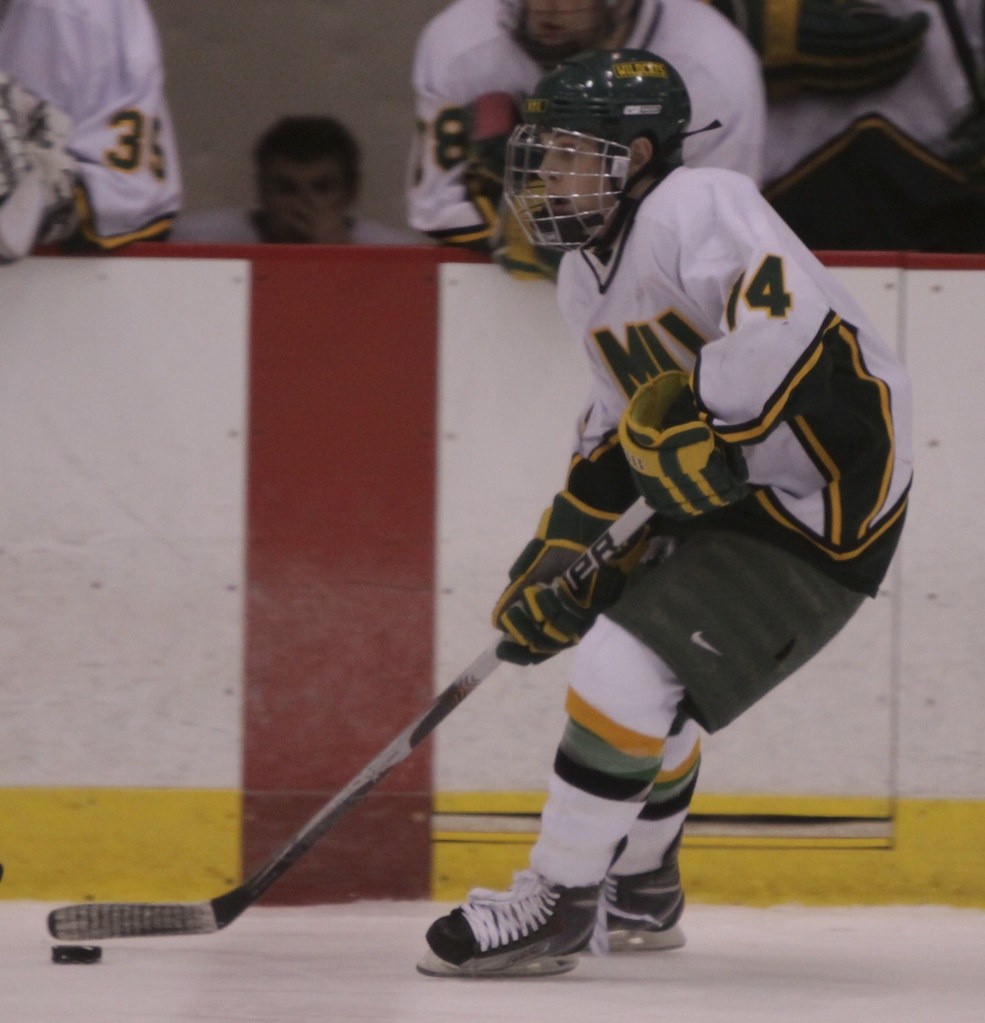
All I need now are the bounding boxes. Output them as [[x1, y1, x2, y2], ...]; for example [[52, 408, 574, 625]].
[[0, 0, 183, 259], [413, 45, 912, 977], [408, 0, 985, 257], [250, 113, 361, 246]]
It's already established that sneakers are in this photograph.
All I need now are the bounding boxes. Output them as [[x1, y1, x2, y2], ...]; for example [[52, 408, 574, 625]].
[[417, 868, 606, 975], [580, 825, 688, 954]]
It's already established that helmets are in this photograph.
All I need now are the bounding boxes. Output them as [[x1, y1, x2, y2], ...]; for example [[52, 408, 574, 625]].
[[502, 46, 692, 250]]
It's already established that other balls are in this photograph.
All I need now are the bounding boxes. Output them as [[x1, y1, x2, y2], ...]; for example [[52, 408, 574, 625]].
[[48, 944, 104, 966]]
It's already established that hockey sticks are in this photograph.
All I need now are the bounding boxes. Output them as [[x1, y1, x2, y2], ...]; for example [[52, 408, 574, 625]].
[[46, 497, 658, 940]]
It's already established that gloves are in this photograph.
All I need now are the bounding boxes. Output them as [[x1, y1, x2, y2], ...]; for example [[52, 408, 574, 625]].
[[491, 488, 643, 666], [620, 370, 747, 519], [709, 0, 931, 101]]
